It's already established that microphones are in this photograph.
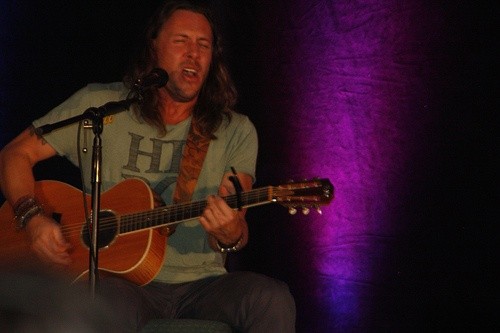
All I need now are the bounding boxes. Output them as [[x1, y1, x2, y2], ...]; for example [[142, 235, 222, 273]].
[[134, 68, 168, 89]]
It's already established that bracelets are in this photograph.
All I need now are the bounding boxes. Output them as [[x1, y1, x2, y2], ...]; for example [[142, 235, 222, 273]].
[[215, 234, 243, 252], [10, 197, 42, 227]]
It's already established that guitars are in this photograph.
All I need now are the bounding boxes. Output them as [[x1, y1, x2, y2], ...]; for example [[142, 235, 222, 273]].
[[0, 175, 335, 302]]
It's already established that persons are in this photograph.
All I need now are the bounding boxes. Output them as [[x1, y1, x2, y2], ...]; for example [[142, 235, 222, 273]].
[[0, 0, 298, 333]]
